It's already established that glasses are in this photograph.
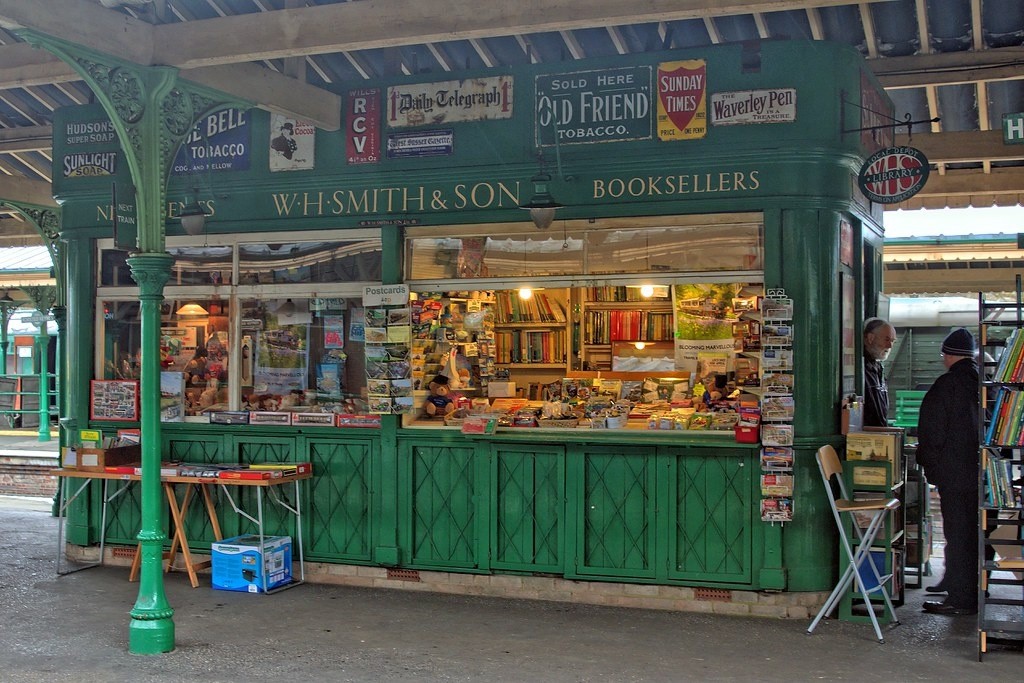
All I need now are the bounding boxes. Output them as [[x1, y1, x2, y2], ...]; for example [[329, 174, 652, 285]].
[[876, 372, 888, 393], [940, 352, 945, 356]]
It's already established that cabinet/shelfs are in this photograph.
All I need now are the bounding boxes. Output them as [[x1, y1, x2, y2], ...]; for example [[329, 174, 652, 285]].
[[838, 452, 908, 630], [405, 297, 496, 428], [493, 288, 571, 379], [580, 286, 676, 370], [978, 289, 1024, 663]]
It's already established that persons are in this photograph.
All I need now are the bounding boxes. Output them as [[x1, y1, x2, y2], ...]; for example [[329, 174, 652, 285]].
[[925, 349, 999, 600], [862, 316, 900, 429], [914, 327, 980, 614]]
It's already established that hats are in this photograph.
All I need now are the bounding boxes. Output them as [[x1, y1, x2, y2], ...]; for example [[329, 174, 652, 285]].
[[942, 328, 974, 357]]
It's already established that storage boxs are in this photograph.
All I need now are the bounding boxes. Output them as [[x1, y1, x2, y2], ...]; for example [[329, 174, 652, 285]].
[[208, 532, 294, 593]]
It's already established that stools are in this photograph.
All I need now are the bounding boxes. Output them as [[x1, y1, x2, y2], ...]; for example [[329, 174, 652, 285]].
[[804, 444, 899, 646]]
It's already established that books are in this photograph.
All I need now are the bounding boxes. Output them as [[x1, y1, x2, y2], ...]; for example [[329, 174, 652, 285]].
[[985, 389, 1024, 446], [993, 326, 1024, 384], [993, 555, 1024, 569], [492, 288, 567, 364], [896, 441, 932, 564], [583, 285, 673, 370], [847, 424, 903, 600], [986, 456, 1020, 509]]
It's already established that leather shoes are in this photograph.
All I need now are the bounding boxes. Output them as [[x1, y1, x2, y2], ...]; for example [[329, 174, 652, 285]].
[[923, 600, 971, 615], [925, 581, 947, 592]]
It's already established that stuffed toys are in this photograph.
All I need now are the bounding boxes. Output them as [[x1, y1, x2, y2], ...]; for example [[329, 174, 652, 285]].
[[240, 389, 306, 411], [699, 375, 729, 413], [422, 373, 455, 416], [182, 356, 225, 407]]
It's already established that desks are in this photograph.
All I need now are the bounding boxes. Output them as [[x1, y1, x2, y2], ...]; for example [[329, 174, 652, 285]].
[[50, 458, 314, 595]]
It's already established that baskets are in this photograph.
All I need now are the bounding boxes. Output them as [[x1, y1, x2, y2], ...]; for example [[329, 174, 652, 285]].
[[444, 407, 498, 427], [536, 411, 585, 428]]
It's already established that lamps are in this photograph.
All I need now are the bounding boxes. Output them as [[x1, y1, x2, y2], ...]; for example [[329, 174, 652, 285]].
[[177, 189, 207, 236], [517, 104, 578, 229], [176, 300, 208, 315]]
